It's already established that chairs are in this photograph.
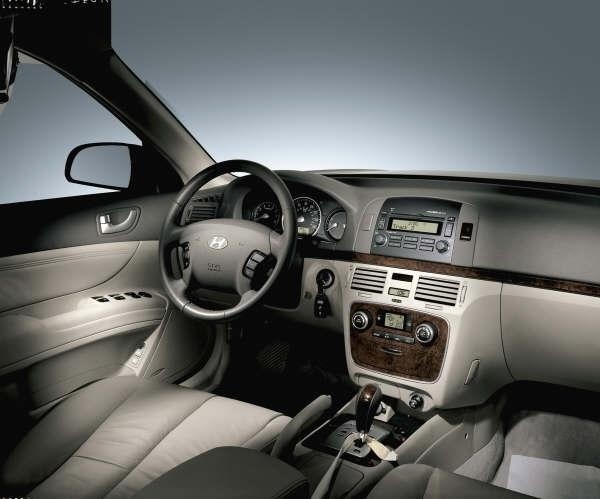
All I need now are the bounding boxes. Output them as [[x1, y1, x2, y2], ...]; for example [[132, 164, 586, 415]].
[[369, 462, 537, 499], [5, 377, 292, 497]]
